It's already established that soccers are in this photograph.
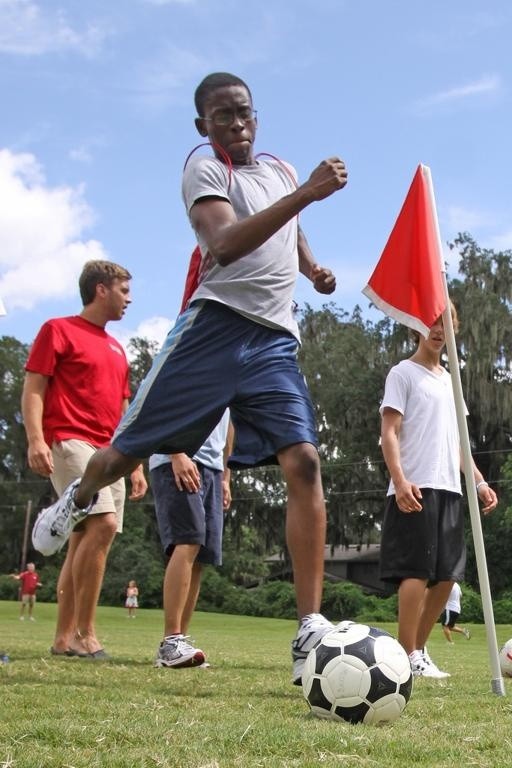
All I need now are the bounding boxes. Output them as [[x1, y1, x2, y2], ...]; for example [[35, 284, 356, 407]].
[[301, 623, 413, 728]]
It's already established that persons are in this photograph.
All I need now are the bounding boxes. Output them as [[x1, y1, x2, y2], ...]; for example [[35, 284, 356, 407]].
[[379, 300, 497, 680], [441, 583, 470, 647], [21, 261, 147, 657], [125, 580, 139, 621], [10, 562, 43, 624], [31, 67, 348, 685], [148, 399, 236, 669]]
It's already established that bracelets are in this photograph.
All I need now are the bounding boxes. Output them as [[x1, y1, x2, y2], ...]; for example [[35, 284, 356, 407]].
[[476, 481, 489, 497]]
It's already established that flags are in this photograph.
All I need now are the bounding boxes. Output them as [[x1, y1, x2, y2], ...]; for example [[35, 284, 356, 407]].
[[360, 167, 446, 337]]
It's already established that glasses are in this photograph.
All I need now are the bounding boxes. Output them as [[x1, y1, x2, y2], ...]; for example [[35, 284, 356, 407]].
[[196, 110, 258, 127]]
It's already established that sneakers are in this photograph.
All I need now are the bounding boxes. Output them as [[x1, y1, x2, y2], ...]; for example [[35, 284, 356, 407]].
[[463, 627, 471, 641], [291, 611, 339, 688], [29, 478, 99, 558], [154, 633, 211, 668], [446, 641, 454, 645], [404, 647, 452, 680]]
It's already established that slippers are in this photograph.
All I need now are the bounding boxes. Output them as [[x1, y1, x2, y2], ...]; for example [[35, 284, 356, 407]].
[[64, 645, 111, 660], [47, 646, 67, 656]]
